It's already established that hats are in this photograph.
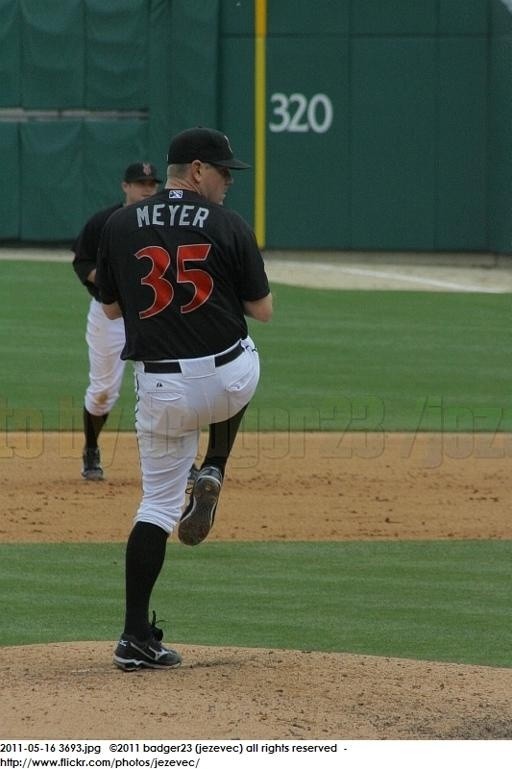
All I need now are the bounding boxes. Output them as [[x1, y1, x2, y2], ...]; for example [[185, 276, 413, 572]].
[[123, 161, 164, 185], [165, 124, 253, 171]]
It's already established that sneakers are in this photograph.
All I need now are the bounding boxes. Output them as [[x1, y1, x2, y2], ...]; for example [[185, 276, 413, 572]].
[[112, 622, 184, 673], [80, 443, 104, 481], [175, 464, 223, 546]]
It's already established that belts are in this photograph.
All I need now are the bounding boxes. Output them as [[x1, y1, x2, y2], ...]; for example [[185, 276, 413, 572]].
[[143, 340, 246, 374]]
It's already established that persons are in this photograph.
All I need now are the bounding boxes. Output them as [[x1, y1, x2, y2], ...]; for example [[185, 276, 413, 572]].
[[92, 123, 274, 675], [68, 160, 165, 484]]
